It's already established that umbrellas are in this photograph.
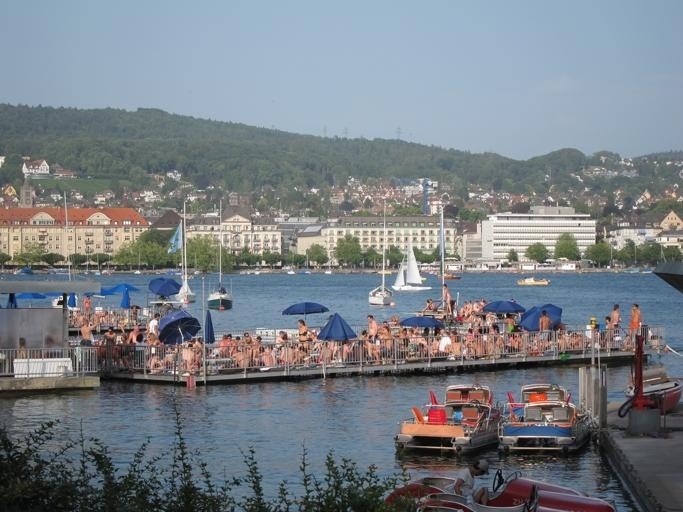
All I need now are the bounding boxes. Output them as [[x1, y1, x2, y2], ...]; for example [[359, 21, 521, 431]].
[[83, 288, 116, 306], [111, 283, 139, 296], [67, 292, 77, 321], [146, 276, 184, 298], [16, 292, 45, 309], [120, 287, 131, 311]]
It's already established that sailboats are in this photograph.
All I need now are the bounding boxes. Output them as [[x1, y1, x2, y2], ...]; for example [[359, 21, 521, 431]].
[[366, 194, 470, 321], [52, 189, 236, 323]]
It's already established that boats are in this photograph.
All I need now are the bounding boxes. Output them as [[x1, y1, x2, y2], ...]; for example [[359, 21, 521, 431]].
[[238, 267, 342, 276], [382, 383, 617, 512], [622, 356, 681, 416], [515, 276, 551, 288]]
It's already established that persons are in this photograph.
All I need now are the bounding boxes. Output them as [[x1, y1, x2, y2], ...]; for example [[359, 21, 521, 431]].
[[10, 301, 669, 377], [451, 457, 489, 506], [439, 282, 453, 316], [354, 300, 640, 367]]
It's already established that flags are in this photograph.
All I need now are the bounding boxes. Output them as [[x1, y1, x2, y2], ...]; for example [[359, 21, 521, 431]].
[[166, 221, 183, 255]]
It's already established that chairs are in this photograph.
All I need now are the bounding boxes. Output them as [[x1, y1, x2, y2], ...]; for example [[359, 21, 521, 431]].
[[436, 405, 478, 422], [524, 405, 569, 422]]
